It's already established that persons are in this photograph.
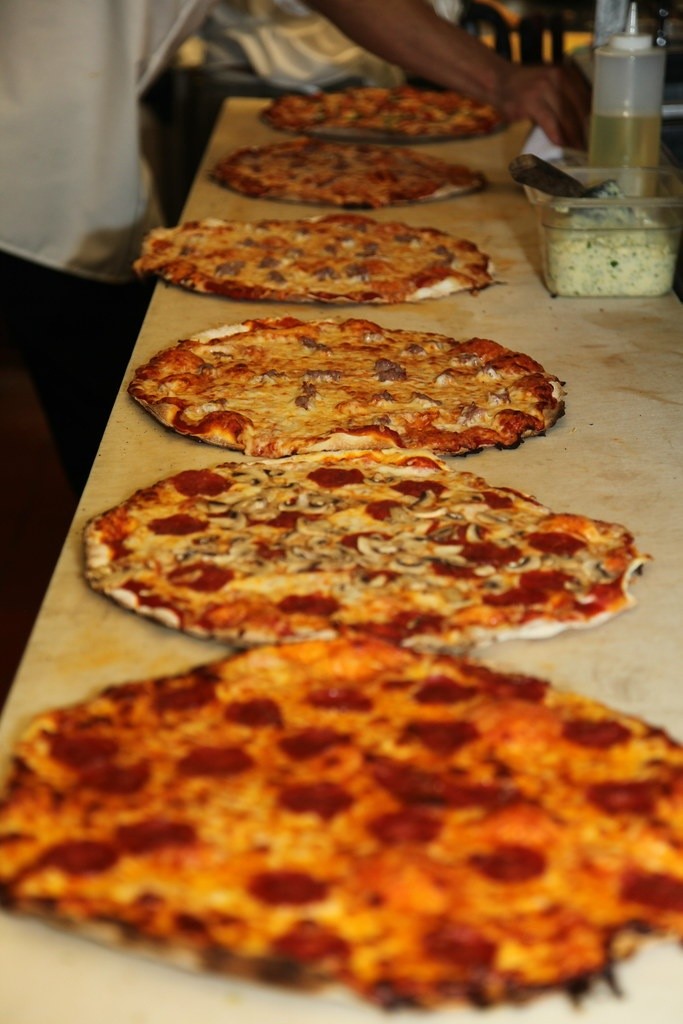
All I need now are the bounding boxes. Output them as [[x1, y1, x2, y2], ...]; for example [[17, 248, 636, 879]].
[[0, 0, 591, 505]]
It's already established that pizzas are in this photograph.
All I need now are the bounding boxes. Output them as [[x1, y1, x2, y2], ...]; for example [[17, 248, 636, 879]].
[[0, 86, 681, 1010]]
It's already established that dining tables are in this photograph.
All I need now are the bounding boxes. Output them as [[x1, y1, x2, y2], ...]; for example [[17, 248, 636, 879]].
[[1, 100, 683, 1022]]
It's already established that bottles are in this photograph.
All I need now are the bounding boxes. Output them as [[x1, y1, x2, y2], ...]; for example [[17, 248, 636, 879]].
[[588, 4, 665, 179]]
